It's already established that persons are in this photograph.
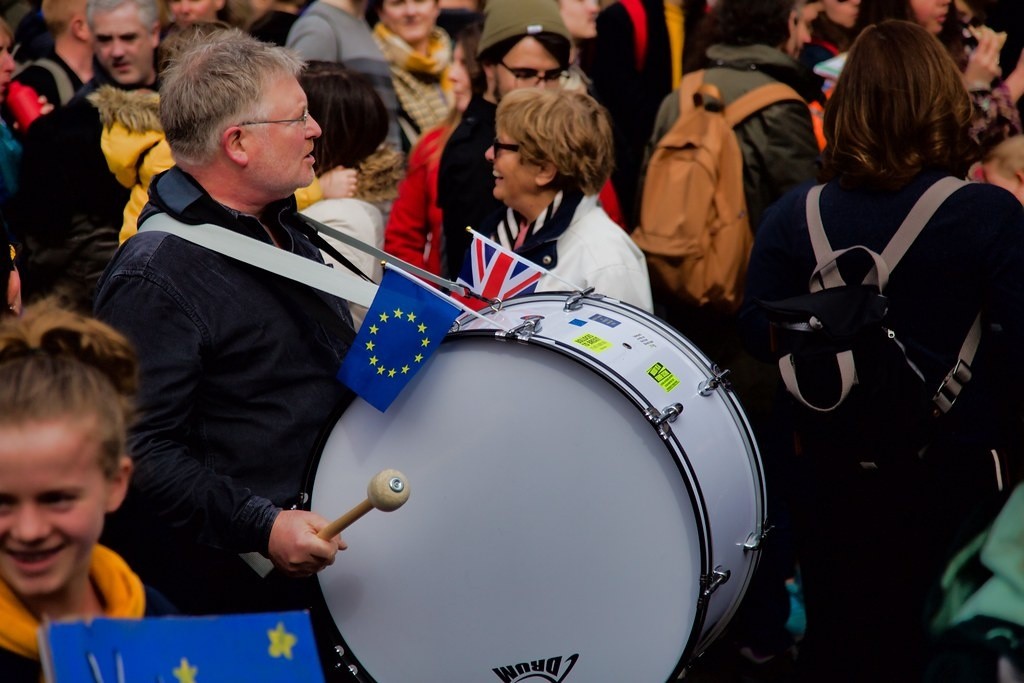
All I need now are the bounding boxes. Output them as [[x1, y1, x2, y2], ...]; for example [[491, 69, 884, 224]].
[[0, 354, 178, 682], [0, 0, 1024, 683]]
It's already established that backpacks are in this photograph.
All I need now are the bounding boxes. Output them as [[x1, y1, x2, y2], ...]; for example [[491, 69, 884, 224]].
[[630, 68, 805, 310], [746, 176, 982, 526]]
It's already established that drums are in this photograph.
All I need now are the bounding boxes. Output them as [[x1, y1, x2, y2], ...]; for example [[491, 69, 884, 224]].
[[307, 287, 776, 683]]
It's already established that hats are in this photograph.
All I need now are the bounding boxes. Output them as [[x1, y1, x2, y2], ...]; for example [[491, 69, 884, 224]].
[[477, 0, 572, 59]]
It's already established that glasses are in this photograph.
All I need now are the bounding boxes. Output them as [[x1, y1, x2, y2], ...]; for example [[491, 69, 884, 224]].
[[493, 138, 520, 158], [496, 57, 570, 91], [237, 107, 308, 127]]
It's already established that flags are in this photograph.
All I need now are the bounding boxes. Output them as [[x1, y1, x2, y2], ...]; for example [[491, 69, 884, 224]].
[[335, 267, 462, 413], [451, 238, 541, 315]]
